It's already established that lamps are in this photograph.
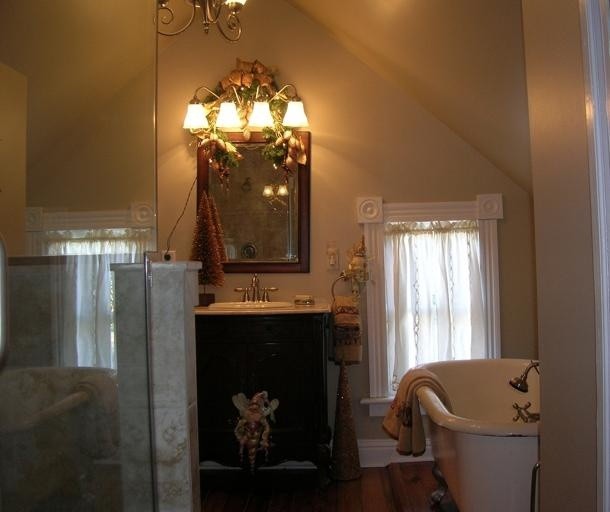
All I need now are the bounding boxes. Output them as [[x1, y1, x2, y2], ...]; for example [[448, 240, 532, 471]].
[[260, 183, 290, 199], [180, 81, 311, 132], [157, 0, 246, 41]]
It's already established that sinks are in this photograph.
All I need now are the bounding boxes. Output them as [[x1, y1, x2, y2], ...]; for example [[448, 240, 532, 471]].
[[208, 301, 293, 309]]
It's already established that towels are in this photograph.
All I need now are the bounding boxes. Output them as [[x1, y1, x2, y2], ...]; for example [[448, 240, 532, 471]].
[[378, 367, 454, 459]]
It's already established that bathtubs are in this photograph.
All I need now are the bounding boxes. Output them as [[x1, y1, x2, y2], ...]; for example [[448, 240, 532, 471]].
[[415, 358, 541, 512]]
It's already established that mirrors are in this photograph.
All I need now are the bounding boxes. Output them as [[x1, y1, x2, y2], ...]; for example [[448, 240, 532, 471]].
[[197, 131, 311, 273], [0, 1, 157, 512]]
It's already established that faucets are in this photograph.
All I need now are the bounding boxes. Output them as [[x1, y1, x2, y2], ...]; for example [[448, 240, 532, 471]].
[[251, 273, 260, 302]]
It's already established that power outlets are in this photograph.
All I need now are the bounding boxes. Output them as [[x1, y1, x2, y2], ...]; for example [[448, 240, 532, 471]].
[[160, 249, 177, 263]]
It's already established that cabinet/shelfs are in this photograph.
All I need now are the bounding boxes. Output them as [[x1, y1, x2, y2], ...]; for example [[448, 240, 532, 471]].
[[191, 313, 331, 512]]
